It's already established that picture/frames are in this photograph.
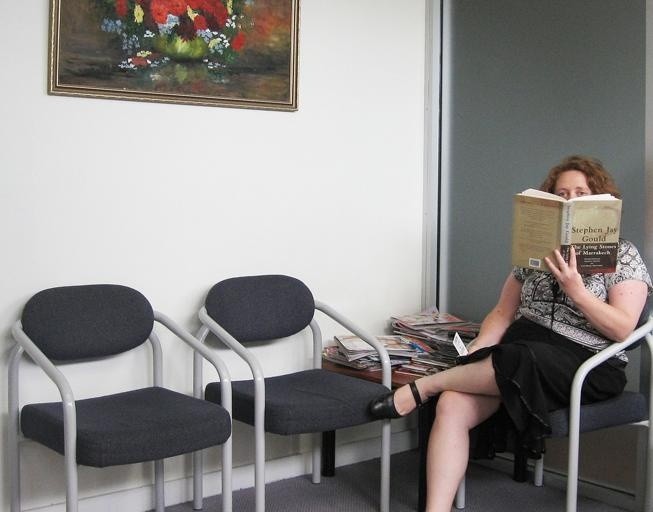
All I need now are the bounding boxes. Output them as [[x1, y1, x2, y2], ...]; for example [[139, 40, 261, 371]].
[[46, 1, 300, 111]]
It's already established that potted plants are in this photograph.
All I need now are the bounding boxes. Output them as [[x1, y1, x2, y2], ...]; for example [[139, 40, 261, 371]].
[[46, 1, 300, 111]]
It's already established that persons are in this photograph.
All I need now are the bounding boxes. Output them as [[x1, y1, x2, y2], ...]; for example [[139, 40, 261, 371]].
[[369, 157, 653, 512]]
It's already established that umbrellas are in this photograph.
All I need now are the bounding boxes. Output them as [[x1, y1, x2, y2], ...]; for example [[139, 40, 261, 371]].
[[367, 381, 424, 419]]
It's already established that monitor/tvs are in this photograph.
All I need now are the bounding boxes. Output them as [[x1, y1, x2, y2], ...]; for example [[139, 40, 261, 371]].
[[322, 309, 482, 376], [512, 188, 623, 273]]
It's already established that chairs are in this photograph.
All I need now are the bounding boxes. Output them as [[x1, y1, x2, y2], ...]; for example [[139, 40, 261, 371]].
[[193, 274, 392, 511], [7, 285, 234, 510], [451, 273, 653, 510]]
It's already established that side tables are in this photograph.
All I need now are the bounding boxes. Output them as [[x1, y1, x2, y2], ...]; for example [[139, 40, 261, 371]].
[[318, 345, 530, 508]]
